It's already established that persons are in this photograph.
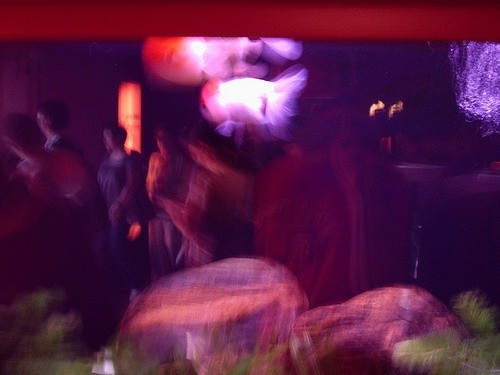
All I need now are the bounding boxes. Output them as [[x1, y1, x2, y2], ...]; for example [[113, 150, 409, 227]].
[[2, 98, 98, 281], [95, 126, 147, 284], [145, 110, 375, 306]]
